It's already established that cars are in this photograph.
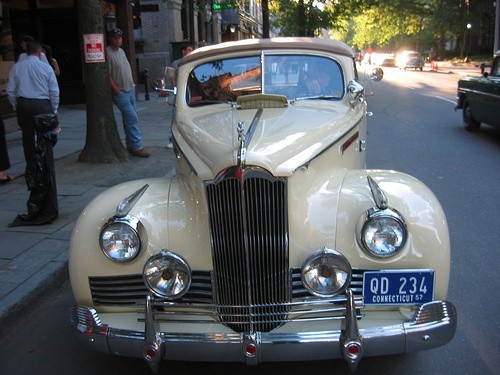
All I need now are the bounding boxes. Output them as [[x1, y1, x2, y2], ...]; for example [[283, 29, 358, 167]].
[[397, 51, 425, 71], [68, 36, 458, 372], [374, 54, 396, 67], [454, 49, 500, 132]]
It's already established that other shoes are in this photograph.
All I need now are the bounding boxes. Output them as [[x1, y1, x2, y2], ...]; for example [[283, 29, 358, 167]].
[[167, 143, 174, 149], [127, 146, 133, 153], [133, 150, 151, 157]]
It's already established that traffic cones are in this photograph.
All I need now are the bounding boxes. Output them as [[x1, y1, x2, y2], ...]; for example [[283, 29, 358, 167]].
[[430, 60, 439, 70]]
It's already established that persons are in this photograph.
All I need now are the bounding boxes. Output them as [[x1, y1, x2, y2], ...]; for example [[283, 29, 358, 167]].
[[166, 44, 194, 149], [302, 70, 334, 96], [0, 115, 15, 182], [429, 48, 435, 61], [205, 70, 239, 100], [106, 28, 150, 157], [7, 35, 60, 190]]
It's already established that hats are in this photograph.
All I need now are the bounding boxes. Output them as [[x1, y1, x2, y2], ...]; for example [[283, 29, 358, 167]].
[[109, 28, 124, 37]]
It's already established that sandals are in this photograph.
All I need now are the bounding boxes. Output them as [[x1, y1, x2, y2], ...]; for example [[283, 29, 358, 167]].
[[0, 175, 14, 183]]
